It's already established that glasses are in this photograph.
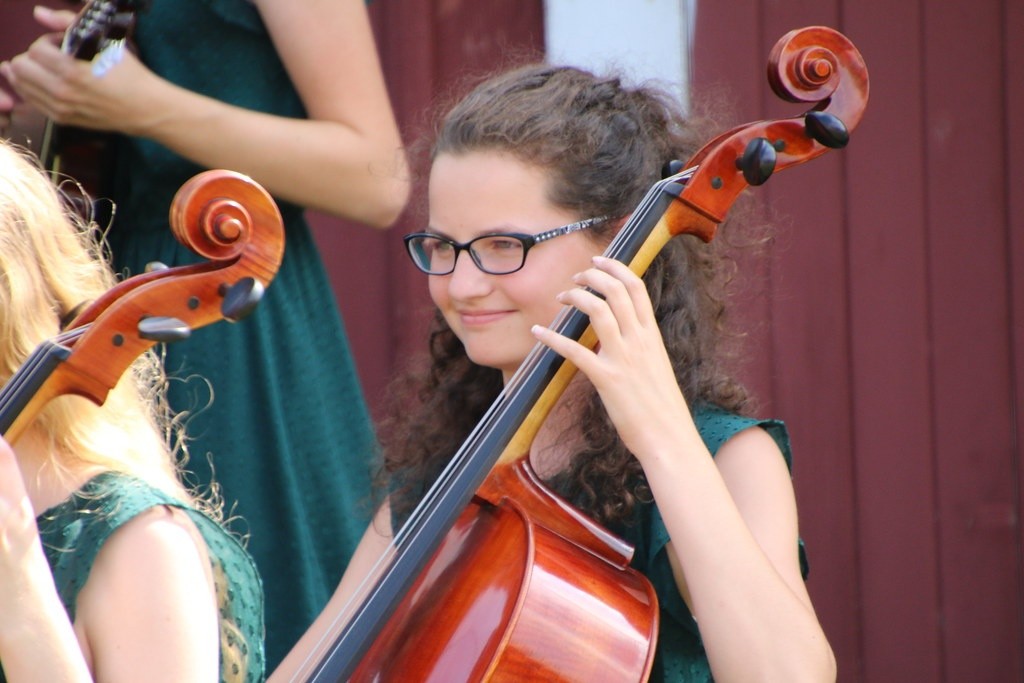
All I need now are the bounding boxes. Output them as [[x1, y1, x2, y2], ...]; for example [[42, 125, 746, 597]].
[[404, 212, 619, 275]]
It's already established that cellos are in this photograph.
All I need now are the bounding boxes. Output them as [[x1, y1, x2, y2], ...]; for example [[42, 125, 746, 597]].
[[36, 0, 138, 171], [0, 169, 284, 453], [284, 28, 868, 683]]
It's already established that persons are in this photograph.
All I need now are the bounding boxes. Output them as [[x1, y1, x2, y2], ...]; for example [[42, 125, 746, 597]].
[[0, 140, 267, 683], [262, 58, 839, 683], [0, 0, 414, 683]]
